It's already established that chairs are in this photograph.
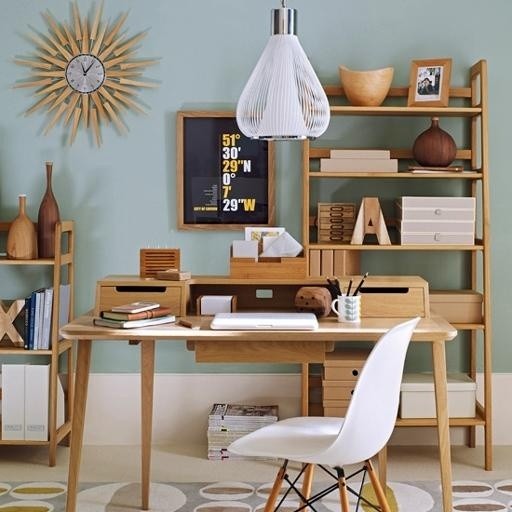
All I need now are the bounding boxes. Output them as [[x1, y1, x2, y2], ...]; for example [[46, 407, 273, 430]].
[[224, 317, 424, 511]]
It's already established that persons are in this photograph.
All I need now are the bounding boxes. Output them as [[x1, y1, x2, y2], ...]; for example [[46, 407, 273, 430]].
[[417, 75, 435, 95]]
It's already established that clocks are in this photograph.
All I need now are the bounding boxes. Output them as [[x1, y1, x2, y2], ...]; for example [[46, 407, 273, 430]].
[[8, 0, 161, 150]]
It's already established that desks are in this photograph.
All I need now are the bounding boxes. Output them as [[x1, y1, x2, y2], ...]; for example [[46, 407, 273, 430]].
[[57, 272, 460, 512]]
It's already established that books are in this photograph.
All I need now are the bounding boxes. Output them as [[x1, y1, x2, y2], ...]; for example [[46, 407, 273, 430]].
[[99, 306, 171, 321], [111, 300, 160, 314], [320, 158, 398, 173], [408, 165, 465, 171], [258, 231, 305, 258], [411, 169, 478, 174], [246, 226, 286, 254], [23, 283, 72, 351], [330, 149, 391, 159], [93, 315, 175, 329], [205, 402, 279, 462], [232, 238, 259, 262]]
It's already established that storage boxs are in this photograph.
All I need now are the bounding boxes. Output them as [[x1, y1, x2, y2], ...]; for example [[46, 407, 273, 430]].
[[428, 289, 484, 325], [319, 350, 478, 420]]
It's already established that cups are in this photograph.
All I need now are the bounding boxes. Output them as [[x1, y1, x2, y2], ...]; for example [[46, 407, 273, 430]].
[[331, 295, 362, 324]]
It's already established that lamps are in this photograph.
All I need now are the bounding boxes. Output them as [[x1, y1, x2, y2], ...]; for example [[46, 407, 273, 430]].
[[233, 0, 331, 142]]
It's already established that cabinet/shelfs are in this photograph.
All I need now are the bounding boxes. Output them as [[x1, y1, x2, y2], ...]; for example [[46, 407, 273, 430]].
[[301, 56, 494, 470], [2, 218, 77, 469]]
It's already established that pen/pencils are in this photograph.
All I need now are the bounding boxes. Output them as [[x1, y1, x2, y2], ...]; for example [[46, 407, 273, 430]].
[[326, 271, 370, 296]]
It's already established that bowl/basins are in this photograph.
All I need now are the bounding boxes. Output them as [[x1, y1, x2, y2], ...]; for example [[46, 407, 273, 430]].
[[338, 65, 394, 106]]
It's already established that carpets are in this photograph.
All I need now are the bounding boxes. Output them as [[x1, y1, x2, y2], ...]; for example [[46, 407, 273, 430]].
[[0, 477, 511, 511]]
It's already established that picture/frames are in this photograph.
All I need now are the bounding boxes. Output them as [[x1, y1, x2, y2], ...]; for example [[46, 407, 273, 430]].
[[407, 58, 453, 108]]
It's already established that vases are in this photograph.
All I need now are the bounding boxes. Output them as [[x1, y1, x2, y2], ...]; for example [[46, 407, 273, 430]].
[[413, 115, 457, 167], [6, 162, 61, 259]]
